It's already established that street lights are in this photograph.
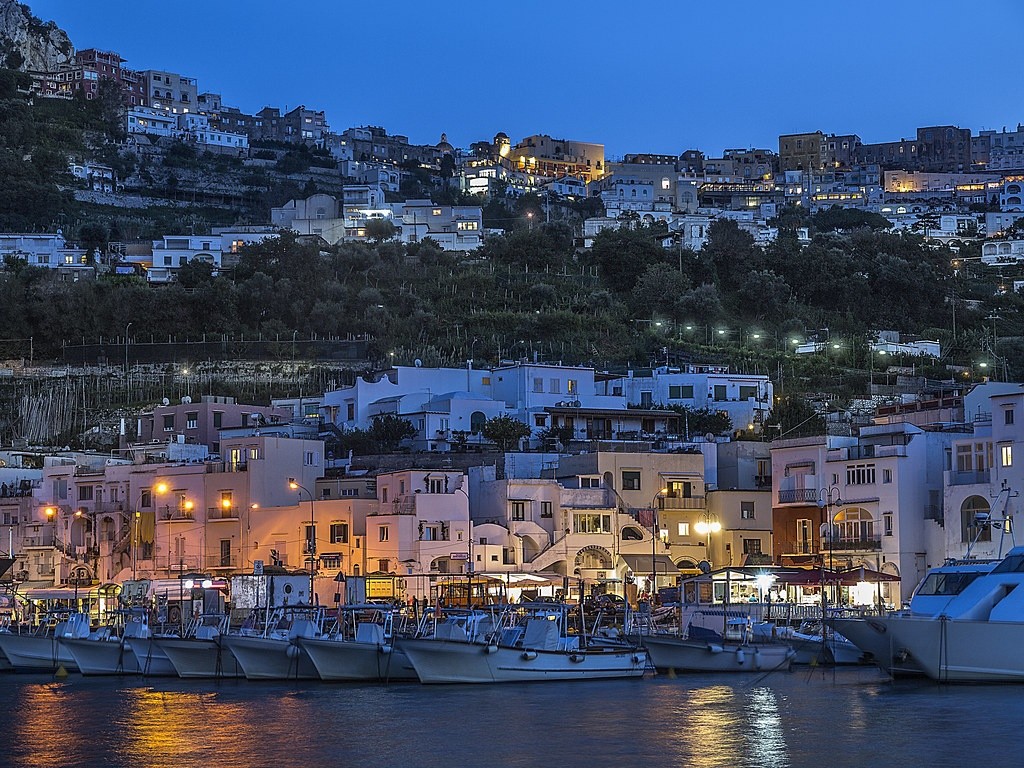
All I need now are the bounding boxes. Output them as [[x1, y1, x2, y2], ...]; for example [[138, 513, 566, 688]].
[[240, 504, 257, 574], [204, 501, 230, 571], [694, 512, 721, 560], [133, 484, 166, 581], [46, 508, 81, 583], [168, 502, 193, 579], [817, 484, 842, 572], [289, 482, 314, 605], [652, 488, 668, 593]]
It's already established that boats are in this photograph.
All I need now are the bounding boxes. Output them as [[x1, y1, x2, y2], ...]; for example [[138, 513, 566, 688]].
[[0, 479, 1023, 686]]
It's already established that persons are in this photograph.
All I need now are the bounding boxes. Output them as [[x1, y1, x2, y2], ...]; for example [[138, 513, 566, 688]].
[[689, 591, 827, 620]]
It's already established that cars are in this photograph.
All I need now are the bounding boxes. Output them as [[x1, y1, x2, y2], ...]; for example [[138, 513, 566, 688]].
[[588, 594, 630, 615]]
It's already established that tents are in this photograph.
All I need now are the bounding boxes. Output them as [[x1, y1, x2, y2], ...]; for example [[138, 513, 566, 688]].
[[774, 569, 902, 616]]
